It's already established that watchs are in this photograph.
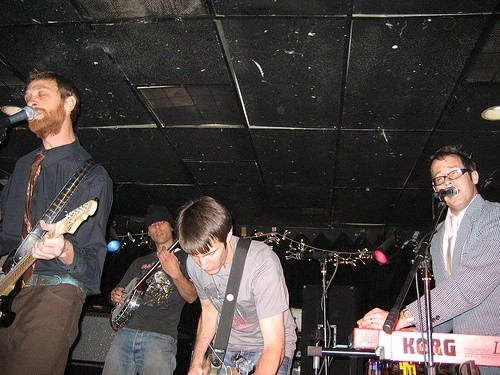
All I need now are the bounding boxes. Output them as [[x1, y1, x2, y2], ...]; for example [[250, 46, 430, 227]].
[[403, 308, 415, 327]]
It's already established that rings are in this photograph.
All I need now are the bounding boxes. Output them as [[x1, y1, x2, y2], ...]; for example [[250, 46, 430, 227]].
[[370, 318, 373, 324]]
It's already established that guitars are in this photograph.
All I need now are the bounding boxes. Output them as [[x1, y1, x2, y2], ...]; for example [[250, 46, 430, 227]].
[[109, 236, 180, 333], [198, 349, 254, 374], [0, 197, 105, 325]]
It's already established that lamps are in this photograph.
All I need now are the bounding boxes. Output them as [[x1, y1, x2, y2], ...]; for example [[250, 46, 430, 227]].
[[105, 226, 122, 252], [373, 227, 406, 264]]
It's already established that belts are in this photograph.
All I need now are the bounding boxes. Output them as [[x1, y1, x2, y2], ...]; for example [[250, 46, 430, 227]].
[[21, 274, 88, 298]]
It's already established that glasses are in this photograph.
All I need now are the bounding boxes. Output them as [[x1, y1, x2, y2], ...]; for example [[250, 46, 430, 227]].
[[431, 167, 472, 186]]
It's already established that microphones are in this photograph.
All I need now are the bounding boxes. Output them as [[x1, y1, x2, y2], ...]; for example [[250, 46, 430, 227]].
[[0, 106, 36, 130], [433, 185, 459, 199]]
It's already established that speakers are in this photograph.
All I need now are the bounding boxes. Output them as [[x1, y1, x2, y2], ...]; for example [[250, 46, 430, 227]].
[[68, 310, 117, 368], [301, 284, 363, 375]]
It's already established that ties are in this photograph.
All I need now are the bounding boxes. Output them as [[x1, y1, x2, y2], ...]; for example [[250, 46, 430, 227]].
[[446, 215, 460, 276]]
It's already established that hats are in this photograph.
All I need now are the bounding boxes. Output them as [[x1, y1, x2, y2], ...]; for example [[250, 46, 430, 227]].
[[145, 203, 175, 231]]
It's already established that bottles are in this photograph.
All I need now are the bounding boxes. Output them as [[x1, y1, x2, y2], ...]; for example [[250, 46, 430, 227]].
[[376, 361, 380, 375], [369, 359, 376, 375], [379, 347, 388, 375], [291, 350, 301, 375]]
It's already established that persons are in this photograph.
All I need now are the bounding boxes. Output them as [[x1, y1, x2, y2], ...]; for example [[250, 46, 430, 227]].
[[0, 68, 113, 375], [174, 196, 298, 375], [101, 202, 197, 375], [357, 145, 500, 375]]
